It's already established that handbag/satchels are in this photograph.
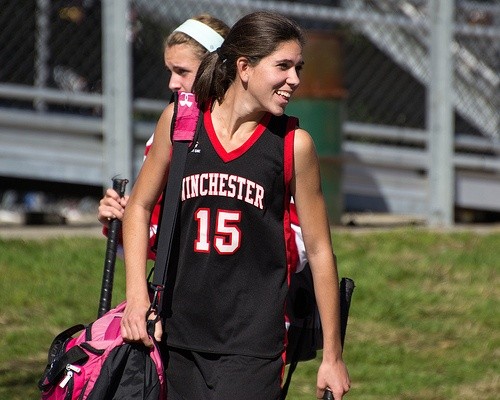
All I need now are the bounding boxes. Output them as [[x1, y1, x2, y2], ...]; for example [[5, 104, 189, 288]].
[[46, 301, 162, 373], [40, 332, 165, 400], [284, 255, 335, 365]]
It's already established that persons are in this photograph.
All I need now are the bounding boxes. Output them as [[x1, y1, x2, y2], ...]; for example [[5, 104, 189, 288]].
[[121, 11, 352, 400], [98, 14, 309, 273]]
